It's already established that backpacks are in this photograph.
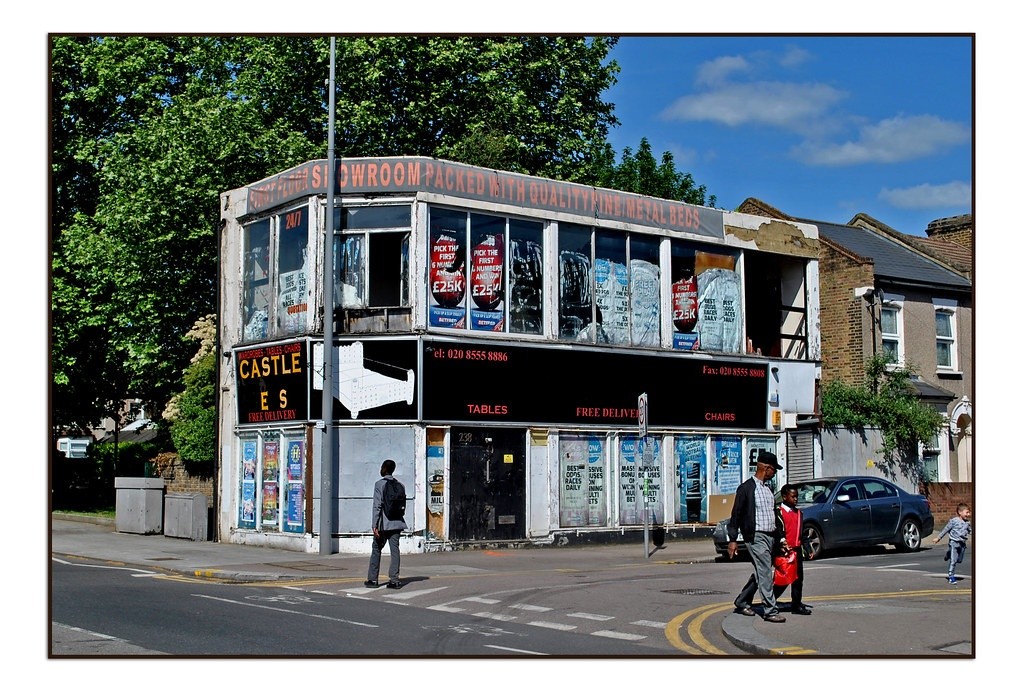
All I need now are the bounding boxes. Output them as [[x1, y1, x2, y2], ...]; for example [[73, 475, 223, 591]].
[[382, 478, 406, 520]]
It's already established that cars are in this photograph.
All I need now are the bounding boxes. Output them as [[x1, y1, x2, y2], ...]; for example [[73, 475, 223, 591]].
[[712, 474, 935, 561]]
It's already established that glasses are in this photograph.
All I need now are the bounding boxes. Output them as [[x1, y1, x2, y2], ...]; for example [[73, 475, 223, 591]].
[[765, 466, 777, 474]]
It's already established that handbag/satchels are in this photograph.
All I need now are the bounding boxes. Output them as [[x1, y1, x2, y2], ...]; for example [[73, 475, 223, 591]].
[[773, 551, 798, 587]]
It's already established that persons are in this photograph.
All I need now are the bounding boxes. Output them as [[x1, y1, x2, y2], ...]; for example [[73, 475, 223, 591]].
[[763, 483, 813, 615], [932, 501, 972, 584], [726, 451, 789, 623], [364, 459, 408, 589]]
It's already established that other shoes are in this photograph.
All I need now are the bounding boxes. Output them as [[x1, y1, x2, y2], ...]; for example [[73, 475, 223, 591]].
[[949, 577, 957, 585], [387, 580, 401, 589], [791, 607, 812, 614], [734, 602, 755, 615], [764, 615, 786, 622], [944, 550, 951, 561], [364, 580, 378, 587]]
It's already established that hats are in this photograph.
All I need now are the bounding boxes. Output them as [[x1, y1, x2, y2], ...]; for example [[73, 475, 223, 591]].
[[757, 452, 783, 470]]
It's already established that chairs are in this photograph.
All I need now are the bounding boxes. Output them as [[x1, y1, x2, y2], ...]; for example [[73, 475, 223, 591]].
[[812, 490, 826, 504], [873, 490, 888, 498], [847, 487, 858, 501], [866, 491, 872, 499]]
